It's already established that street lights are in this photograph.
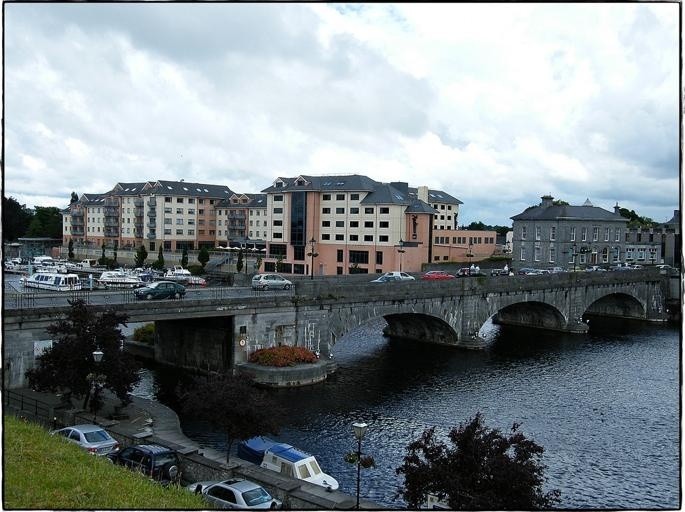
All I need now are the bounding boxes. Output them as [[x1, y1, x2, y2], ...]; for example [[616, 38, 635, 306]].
[[506, 241, 510, 258], [352, 417, 368, 509], [399, 239, 403, 272], [573, 242, 577, 272], [92, 345, 103, 421], [469, 243, 473, 276], [309, 238, 316, 280], [244, 236, 250, 275]]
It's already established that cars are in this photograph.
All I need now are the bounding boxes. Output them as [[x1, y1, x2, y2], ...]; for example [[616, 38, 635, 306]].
[[50, 424, 121, 457], [370, 271, 415, 283], [656, 265, 673, 269], [491, 269, 503, 277], [518, 267, 567, 276], [456, 268, 487, 278], [421, 271, 455, 280], [251, 274, 293, 290], [189, 477, 282, 510], [134, 282, 187, 300], [586, 263, 643, 273], [70, 259, 107, 272]]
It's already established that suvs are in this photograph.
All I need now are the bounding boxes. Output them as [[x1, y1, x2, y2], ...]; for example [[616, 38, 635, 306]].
[[106, 443, 182, 488]]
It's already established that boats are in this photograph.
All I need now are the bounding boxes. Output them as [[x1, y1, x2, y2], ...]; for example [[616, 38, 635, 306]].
[[238, 435, 340, 491], [4, 254, 69, 274], [20, 272, 82, 292], [83, 266, 207, 289]]
